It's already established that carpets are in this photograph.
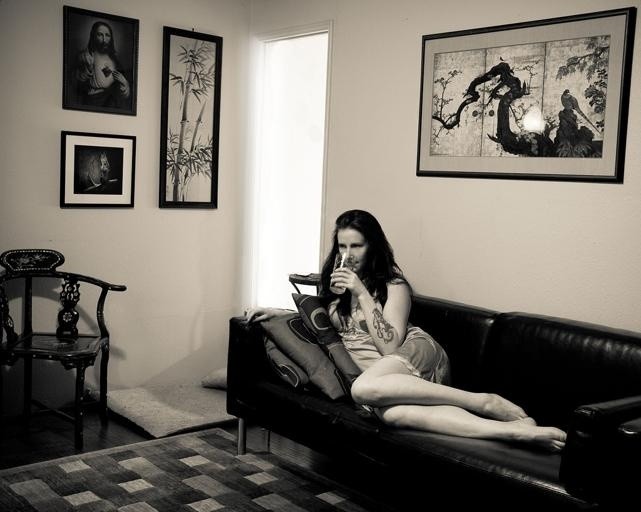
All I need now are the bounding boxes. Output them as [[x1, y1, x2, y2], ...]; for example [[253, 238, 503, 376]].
[[87, 373, 237, 440], [0, 425, 384, 512]]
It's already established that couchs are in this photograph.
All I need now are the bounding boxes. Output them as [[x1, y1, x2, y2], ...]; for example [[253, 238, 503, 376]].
[[223, 292, 639, 512]]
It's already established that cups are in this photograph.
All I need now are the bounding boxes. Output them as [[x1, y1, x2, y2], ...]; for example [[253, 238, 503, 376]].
[[329, 253, 355, 294]]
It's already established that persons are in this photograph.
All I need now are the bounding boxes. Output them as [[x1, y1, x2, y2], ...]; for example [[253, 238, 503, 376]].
[[70, 17, 134, 106], [244, 209, 571, 455], [556, 101, 579, 139], [99, 149, 122, 194]]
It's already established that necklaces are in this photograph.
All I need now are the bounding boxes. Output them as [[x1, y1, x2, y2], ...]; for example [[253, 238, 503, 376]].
[[93, 51, 110, 67]]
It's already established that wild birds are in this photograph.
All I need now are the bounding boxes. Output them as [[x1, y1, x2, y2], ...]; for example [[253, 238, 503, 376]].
[[561, 89, 600, 133]]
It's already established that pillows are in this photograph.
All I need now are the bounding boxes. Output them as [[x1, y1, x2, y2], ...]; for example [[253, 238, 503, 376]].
[[200, 367, 229, 387], [259, 293, 364, 401]]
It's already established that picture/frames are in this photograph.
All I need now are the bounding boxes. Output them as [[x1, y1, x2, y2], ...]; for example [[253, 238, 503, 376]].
[[60, 127, 136, 210], [61, 4, 141, 117], [414, 6, 637, 190], [157, 25, 225, 210]]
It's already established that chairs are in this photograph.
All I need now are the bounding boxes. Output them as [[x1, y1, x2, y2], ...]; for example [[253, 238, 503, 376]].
[[2, 247, 126, 453]]
